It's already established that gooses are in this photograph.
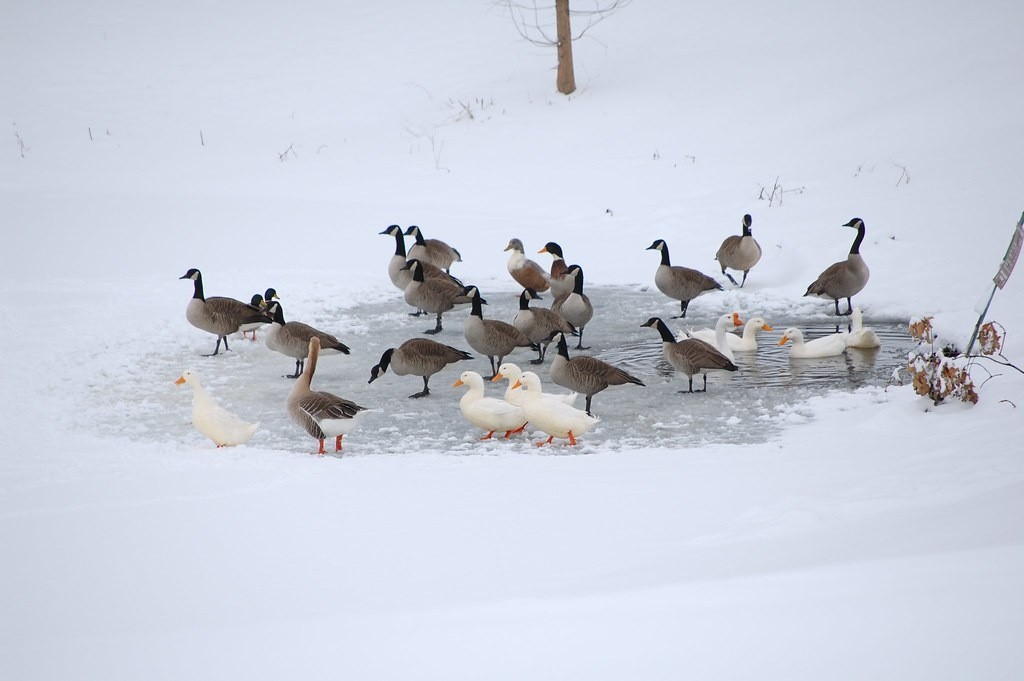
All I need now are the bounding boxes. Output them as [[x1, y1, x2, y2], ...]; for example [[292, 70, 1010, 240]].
[[777, 327, 849, 359], [676, 313, 772, 366], [849, 308, 881, 348], [451, 362, 603, 449], [175, 370, 260, 449]]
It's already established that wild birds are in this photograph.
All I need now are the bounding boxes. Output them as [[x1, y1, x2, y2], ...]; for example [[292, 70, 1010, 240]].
[[714, 214, 762, 288], [503, 238, 594, 365], [368, 338, 474, 399], [646, 239, 724, 317], [254, 300, 351, 380], [456, 285, 524, 381], [803, 217, 871, 316], [178, 269, 274, 359], [284, 337, 367, 452], [638, 316, 738, 393], [540, 329, 647, 418], [378, 224, 490, 335], [241, 288, 286, 342]]
